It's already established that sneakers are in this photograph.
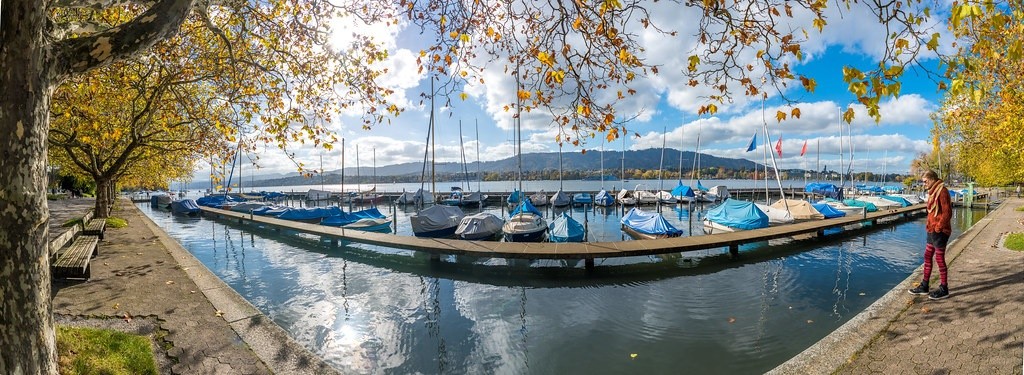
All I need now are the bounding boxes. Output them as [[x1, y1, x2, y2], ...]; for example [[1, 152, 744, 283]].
[[908, 280, 929, 295], [929, 285, 950, 300]]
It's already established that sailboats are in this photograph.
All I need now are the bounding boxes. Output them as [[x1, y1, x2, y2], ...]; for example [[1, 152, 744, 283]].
[[170, 181, 191, 198], [195, 73, 924, 241]]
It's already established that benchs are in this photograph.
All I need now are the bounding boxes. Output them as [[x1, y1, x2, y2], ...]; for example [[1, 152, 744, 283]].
[[82, 210, 106, 241], [46, 223, 99, 284]]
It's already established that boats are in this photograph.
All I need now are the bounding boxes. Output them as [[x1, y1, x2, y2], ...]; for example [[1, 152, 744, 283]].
[[152, 193, 173, 209], [173, 199, 201, 214]]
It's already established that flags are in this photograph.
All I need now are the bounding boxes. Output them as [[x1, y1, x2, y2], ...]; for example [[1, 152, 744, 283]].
[[801, 141, 807, 156], [775, 137, 782, 157], [746, 133, 757, 152]]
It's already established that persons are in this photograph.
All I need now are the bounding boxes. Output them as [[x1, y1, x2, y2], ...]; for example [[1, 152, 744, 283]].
[[1017, 183, 1021, 199], [908, 169, 952, 300], [51, 186, 83, 198]]
